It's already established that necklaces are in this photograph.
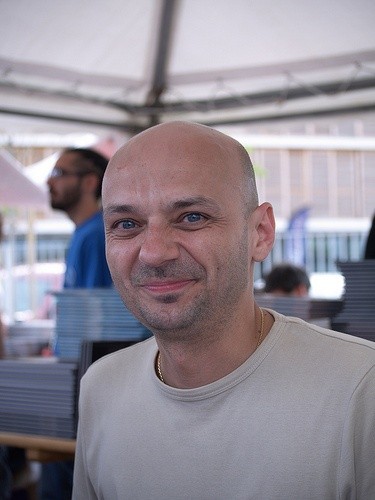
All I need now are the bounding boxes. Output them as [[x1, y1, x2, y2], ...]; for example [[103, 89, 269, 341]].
[[157, 302, 265, 389]]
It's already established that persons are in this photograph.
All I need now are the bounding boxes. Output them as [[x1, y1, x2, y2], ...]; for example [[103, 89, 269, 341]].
[[253, 263, 311, 297], [71, 121, 375, 500], [36, 146, 136, 500]]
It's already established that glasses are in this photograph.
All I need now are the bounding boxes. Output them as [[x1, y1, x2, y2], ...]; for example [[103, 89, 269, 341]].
[[50, 167, 88, 178]]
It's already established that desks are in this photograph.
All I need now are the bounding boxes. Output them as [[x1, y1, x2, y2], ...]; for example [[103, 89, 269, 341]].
[[0, 432, 76, 500]]
